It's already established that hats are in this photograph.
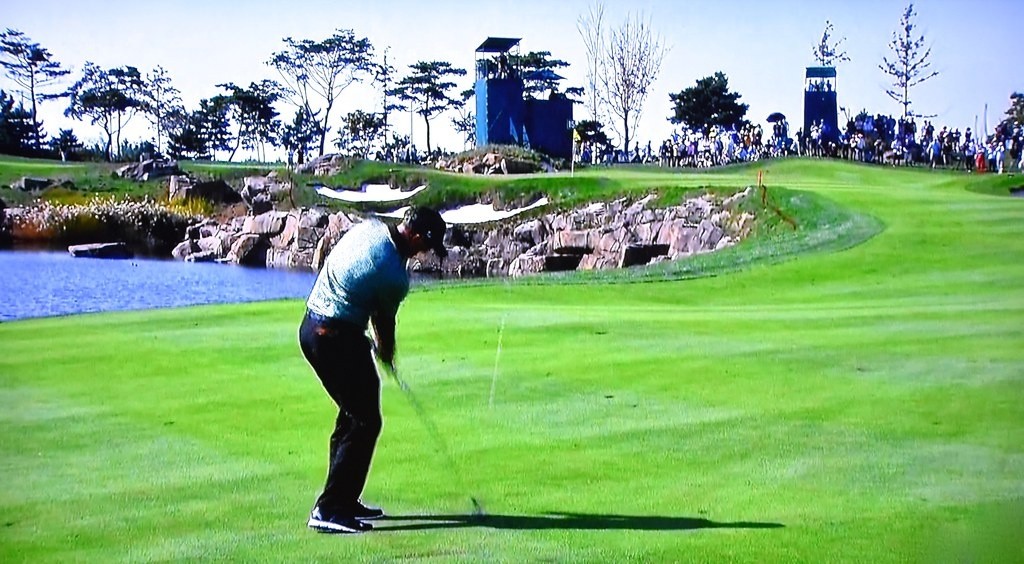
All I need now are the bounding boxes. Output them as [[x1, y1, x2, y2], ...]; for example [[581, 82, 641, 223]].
[[404, 207, 448, 258]]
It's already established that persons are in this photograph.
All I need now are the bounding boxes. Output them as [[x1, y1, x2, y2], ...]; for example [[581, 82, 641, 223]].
[[574, 139, 656, 166], [298, 147, 304, 164], [375, 145, 422, 166], [838, 113, 1024, 175], [299, 205, 448, 534], [808, 78, 832, 92], [287, 149, 294, 164], [658, 118, 836, 168]]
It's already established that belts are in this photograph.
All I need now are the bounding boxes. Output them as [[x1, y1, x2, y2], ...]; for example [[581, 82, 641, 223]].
[[305, 311, 341, 324]]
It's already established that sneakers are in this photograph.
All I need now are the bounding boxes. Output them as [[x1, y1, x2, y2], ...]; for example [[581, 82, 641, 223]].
[[354, 499, 383, 519], [309, 505, 372, 534]]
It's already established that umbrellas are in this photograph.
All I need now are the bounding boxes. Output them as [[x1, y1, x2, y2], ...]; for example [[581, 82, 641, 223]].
[[766, 113, 785, 122]]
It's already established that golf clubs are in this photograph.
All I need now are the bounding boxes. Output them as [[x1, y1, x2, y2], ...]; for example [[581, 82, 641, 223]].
[[366, 327, 487, 521]]
